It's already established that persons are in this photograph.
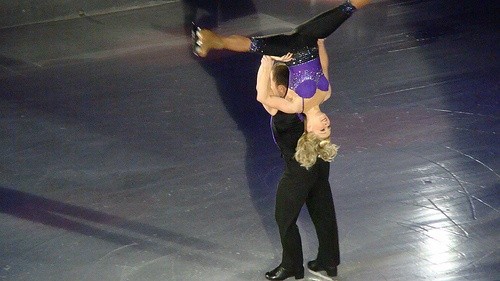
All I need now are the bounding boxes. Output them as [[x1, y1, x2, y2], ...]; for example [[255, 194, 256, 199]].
[[195, 0, 371, 167], [256, 55, 341, 281]]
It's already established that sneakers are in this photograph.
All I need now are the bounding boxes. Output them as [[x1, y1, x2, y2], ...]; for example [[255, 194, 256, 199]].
[[265, 264, 304, 281], [189, 20, 224, 57], [307, 257, 338, 281]]
